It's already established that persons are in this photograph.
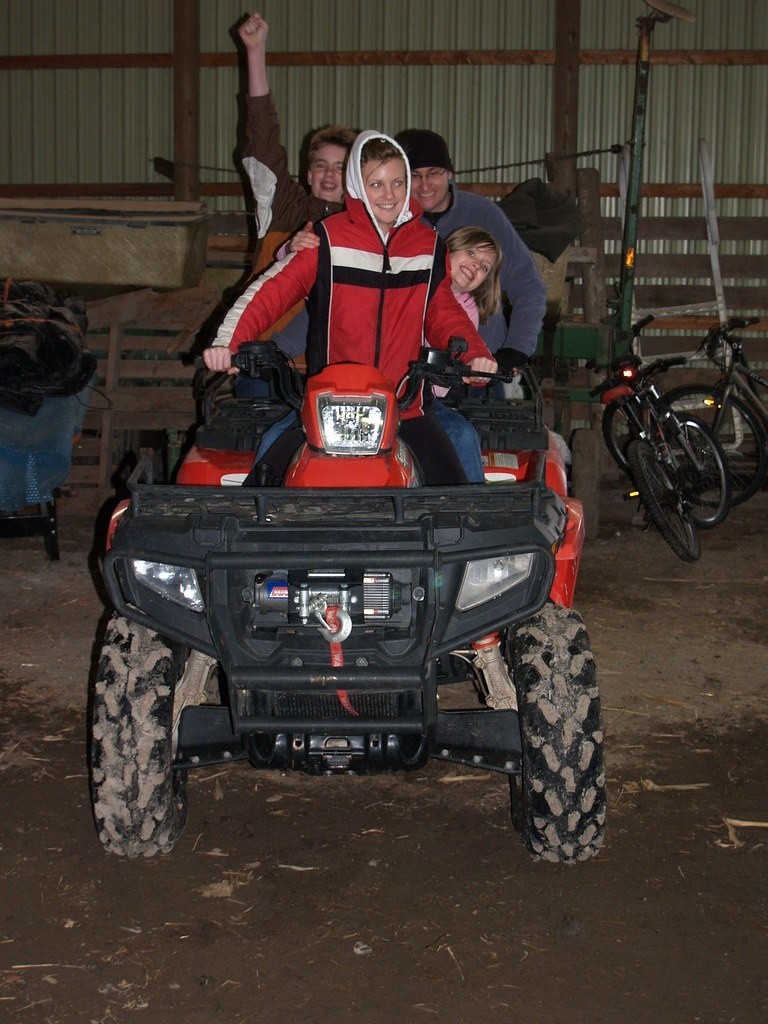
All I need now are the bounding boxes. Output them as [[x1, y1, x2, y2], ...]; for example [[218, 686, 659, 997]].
[[205, 131, 497, 488], [227, 13, 549, 401]]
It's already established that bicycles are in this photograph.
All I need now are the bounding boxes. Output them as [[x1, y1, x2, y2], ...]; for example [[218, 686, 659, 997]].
[[586, 313, 768, 563]]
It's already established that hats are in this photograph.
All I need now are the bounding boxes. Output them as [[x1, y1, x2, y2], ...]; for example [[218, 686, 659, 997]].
[[396, 130, 451, 171]]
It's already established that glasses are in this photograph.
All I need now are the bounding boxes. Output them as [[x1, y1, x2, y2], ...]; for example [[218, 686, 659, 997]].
[[411, 168, 446, 180]]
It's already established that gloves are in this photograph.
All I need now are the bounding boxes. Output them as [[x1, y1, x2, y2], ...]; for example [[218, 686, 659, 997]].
[[495, 347, 528, 371]]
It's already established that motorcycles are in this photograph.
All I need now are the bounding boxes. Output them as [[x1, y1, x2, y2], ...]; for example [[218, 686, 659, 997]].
[[87, 341, 612, 864]]
